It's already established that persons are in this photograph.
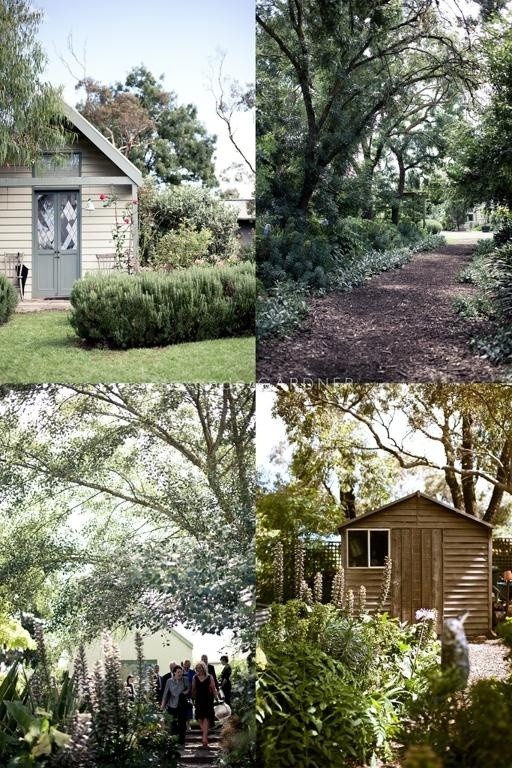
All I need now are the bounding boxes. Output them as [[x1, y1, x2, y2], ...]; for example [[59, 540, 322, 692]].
[[217, 656, 232, 709], [159, 666, 191, 749], [182, 658, 196, 732], [124, 674, 135, 702], [190, 661, 220, 747], [201, 656, 219, 698], [145, 661, 183, 705]]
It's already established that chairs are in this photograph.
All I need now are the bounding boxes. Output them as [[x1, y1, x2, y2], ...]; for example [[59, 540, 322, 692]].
[[95, 252, 117, 272], [0, 250, 25, 302]]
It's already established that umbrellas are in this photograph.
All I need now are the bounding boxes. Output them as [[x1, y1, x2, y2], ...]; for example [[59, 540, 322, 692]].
[[15, 253, 28, 295]]
